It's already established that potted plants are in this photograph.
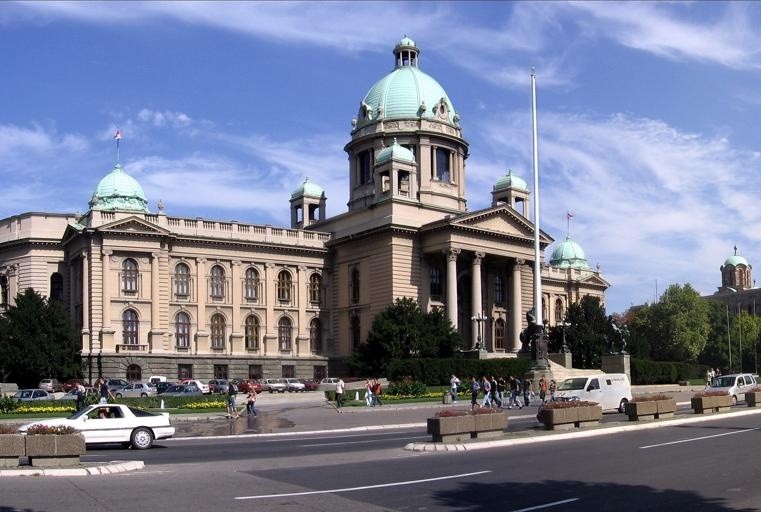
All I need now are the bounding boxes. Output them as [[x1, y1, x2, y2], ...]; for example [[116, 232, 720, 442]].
[[0, 423, 24, 466], [25, 424, 86, 466]]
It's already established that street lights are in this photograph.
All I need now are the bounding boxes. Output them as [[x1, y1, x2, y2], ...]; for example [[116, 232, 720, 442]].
[[528, 65, 546, 325], [555, 320, 572, 353], [472, 312, 488, 349]]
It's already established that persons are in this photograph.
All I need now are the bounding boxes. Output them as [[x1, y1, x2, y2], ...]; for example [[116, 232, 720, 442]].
[[368, 376, 385, 406], [227, 382, 240, 418], [97, 409, 108, 418], [75, 382, 86, 412], [705, 367, 721, 386], [98, 378, 115, 404], [335, 375, 345, 407], [246, 383, 258, 418], [450, 374, 462, 400], [470, 374, 557, 411], [364, 379, 373, 406]]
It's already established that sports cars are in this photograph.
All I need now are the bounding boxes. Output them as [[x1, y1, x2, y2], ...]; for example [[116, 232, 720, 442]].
[[17, 405, 175, 451]]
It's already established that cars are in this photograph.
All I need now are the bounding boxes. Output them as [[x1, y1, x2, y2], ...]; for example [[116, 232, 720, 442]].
[[704, 373, 759, 406]]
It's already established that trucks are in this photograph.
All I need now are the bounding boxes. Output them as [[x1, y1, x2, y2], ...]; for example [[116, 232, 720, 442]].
[[552, 372, 634, 414]]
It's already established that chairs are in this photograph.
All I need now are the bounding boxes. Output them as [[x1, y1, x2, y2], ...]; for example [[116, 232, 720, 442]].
[[107, 412, 115, 418]]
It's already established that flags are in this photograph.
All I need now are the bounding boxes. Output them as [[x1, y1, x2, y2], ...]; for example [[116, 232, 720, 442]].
[[114, 129, 121, 139], [567, 211, 573, 220]]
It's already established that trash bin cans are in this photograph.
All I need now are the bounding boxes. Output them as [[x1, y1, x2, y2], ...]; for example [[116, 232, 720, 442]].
[[443, 390, 452, 404]]
